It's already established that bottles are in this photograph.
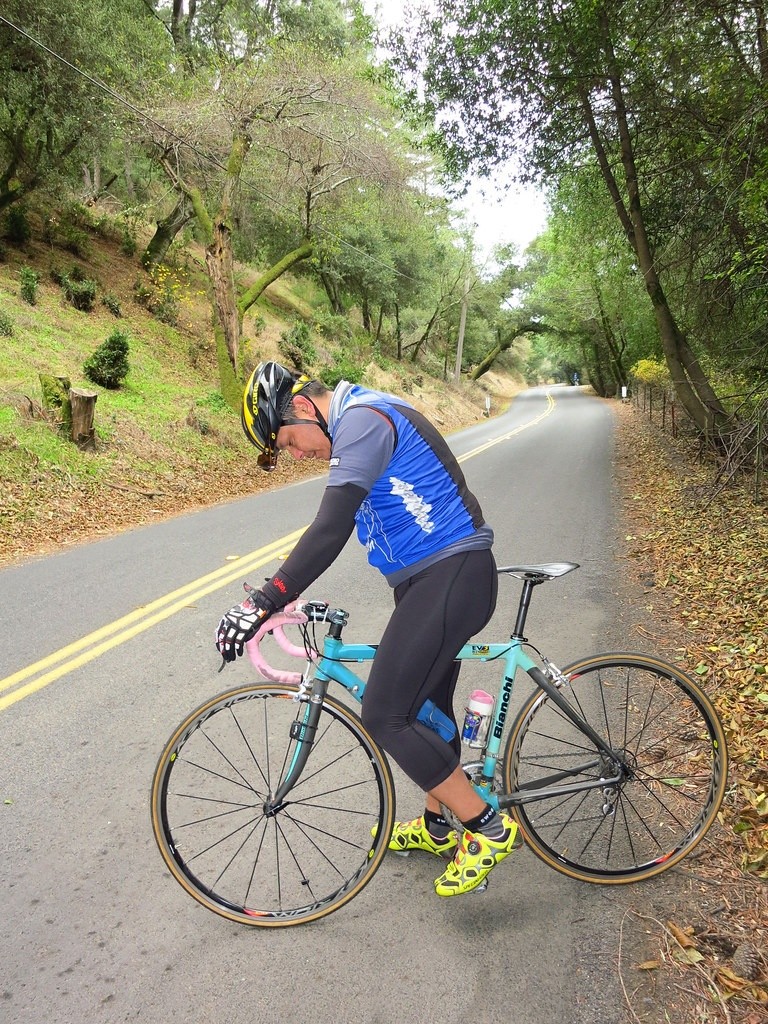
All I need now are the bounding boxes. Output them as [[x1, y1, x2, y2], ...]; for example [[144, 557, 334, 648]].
[[461, 690, 494, 748], [416, 699, 457, 743]]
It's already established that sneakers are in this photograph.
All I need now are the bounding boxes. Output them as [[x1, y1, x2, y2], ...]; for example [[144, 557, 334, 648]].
[[371, 814, 460, 861], [433, 813, 525, 897]]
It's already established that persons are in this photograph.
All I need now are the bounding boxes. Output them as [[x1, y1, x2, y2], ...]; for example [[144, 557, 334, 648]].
[[215, 360, 525, 895]]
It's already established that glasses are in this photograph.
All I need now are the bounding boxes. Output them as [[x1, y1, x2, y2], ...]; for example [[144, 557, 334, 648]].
[[257, 407, 279, 472]]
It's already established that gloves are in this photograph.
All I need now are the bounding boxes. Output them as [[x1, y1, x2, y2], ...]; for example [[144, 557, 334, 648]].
[[213, 582, 277, 663]]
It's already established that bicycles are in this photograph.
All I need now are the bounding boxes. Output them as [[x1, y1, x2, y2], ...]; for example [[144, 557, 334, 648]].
[[150, 562, 730, 931]]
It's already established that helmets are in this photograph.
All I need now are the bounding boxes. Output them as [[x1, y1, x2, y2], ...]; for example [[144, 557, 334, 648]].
[[239, 360, 293, 457]]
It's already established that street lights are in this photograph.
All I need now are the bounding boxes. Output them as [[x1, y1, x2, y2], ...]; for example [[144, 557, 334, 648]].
[[453, 221, 479, 387]]
